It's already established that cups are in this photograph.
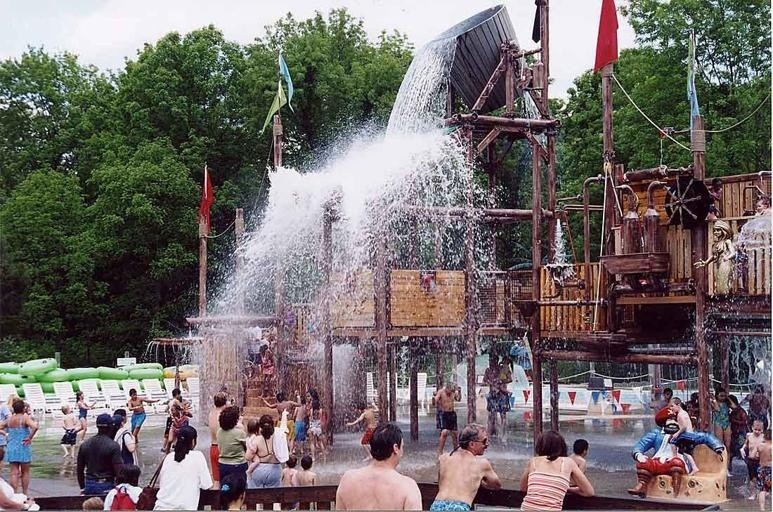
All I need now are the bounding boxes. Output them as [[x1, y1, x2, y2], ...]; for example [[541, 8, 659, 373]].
[[472, 438, 487, 445]]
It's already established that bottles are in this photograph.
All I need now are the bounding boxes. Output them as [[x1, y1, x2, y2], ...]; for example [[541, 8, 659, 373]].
[[96, 414, 115, 426]]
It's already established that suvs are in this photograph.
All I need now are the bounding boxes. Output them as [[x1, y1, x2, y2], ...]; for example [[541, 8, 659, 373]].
[[433, 4, 531, 116]]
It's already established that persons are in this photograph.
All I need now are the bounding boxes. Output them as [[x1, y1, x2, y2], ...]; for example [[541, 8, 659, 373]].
[[627, 404, 725, 498], [431, 382, 462, 459], [259, 347, 276, 399], [209, 392, 243, 485], [567, 439, 589, 487], [497, 356, 513, 399], [104, 464, 143, 510], [2, 393, 34, 460], [75, 391, 96, 443], [113, 409, 138, 468], [756, 196, 770, 216], [2, 401, 38, 495], [647, 384, 773, 511], [282, 455, 300, 487], [153, 425, 214, 510], [127, 388, 160, 444], [60, 404, 81, 458], [161, 388, 193, 453], [709, 178, 723, 200], [693, 220, 735, 297], [219, 474, 246, 511], [246, 409, 289, 488], [707, 206, 717, 220], [483, 355, 509, 443], [429, 423, 502, 512], [245, 419, 260, 479], [217, 406, 251, 490], [520, 431, 594, 512], [77, 414, 123, 495], [258, 389, 327, 465], [295, 456, 318, 487], [346, 401, 380, 462], [336, 423, 422, 511]]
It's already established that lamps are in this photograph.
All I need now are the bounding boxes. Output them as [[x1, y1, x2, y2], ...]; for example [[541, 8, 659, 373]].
[[0, 378, 201, 424], [367, 371, 430, 414]]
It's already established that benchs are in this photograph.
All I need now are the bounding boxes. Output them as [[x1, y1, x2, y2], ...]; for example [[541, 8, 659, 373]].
[[199, 165, 215, 230], [593, 1, 618, 74]]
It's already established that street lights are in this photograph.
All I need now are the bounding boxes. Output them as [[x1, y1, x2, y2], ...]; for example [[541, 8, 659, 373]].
[[136, 462, 162, 510], [121, 432, 133, 464]]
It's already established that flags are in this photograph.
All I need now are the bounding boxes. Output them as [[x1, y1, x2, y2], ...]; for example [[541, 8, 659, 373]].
[[593, 1, 618, 74], [199, 165, 215, 230]]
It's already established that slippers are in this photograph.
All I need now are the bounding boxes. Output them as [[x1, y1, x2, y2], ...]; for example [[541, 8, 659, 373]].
[[111, 486, 135, 512]]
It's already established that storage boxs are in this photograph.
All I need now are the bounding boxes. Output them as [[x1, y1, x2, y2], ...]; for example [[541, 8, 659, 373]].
[[633, 442, 728, 504]]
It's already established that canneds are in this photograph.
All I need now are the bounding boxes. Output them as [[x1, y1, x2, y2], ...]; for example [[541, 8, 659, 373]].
[[0, 357, 200, 396]]
